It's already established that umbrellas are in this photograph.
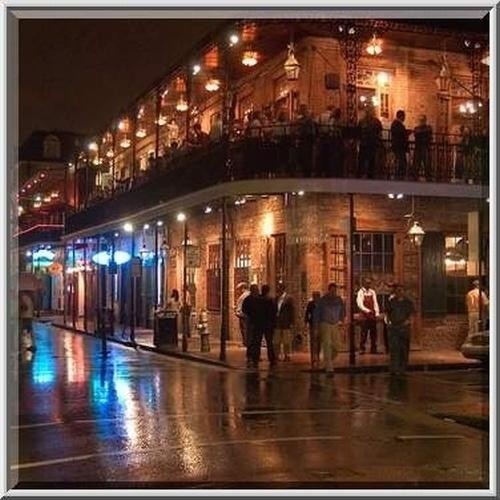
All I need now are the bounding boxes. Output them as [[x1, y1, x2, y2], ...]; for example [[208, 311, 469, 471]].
[[20, 273, 45, 292]]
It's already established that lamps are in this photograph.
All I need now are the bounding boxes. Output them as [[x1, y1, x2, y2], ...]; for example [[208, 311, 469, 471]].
[[282, 45, 303, 82], [433, 63, 453, 92], [138, 233, 192, 266], [404, 220, 427, 248]]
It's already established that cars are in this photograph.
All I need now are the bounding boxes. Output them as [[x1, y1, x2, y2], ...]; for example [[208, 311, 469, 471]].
[[457, 315, 490, 360]]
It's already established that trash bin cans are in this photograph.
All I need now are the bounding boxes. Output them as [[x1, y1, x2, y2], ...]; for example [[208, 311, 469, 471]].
[[92, 307, 114, 337], [153, 309, 179, 349]]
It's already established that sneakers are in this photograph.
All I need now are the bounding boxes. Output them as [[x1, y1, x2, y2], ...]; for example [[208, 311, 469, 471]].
[[326, 371, 335, 378], [27, 346, 37, 351], [359, 350, 365, 355], [244, 354, 291, 370], [370, 350, 383, 354]]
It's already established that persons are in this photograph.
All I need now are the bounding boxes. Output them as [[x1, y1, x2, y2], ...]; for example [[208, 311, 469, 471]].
[[242, 285, 263, 363], [304, 291, 323, 366], [21, 292, 38, 351], [166, 288, 180, 318], [242, 361, 341, 439], [314, 282, 345, 377], [182, 285, 193, 338], [465, 280, 489, 342], [386, 285, 417, 376], [250, 285, 280, 365], [356, 278, 385, 354], [272, 282, 296, 362], [246, 104, 489, 184], [235, 281, 250, 345], [156, 123, 210, 166], [386, 375, 410, 441]]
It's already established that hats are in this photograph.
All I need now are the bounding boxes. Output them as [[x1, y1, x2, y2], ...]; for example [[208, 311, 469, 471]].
[[312, 291, 321, 295], [236, 282, 247, 290]]
